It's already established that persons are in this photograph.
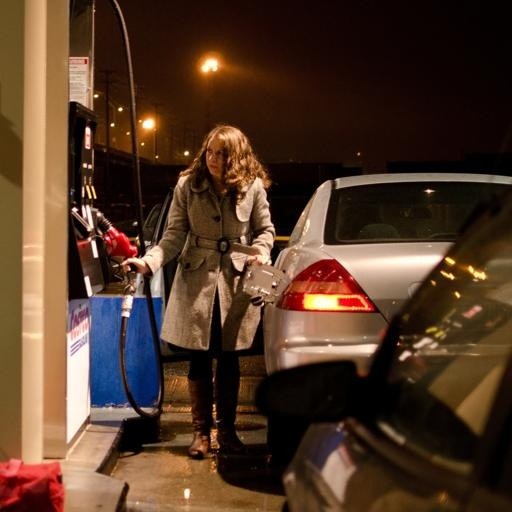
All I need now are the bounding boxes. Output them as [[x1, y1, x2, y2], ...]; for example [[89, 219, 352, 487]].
[[115, 123, 277, 460]]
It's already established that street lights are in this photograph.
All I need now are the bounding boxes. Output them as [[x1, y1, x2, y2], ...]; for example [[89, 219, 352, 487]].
[[90, 90, 124, 128], [199, 58, 218, 138]]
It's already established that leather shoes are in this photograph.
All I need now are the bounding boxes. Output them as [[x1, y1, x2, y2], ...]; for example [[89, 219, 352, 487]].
[[189, 431, 210, 458], [217, 430, 246, 452]]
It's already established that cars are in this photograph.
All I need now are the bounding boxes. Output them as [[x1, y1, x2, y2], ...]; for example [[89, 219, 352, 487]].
[[246, 178, 511, 512], [122, 159, 512, 433], [134, 202, 160, 248], [272, 235, 290, 256]]
